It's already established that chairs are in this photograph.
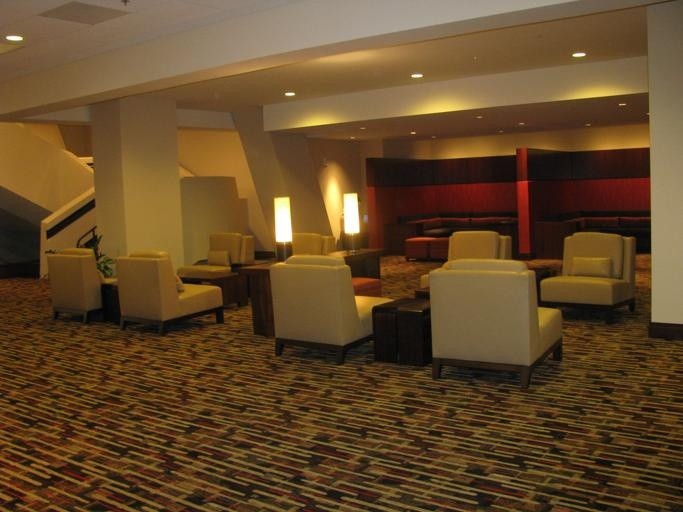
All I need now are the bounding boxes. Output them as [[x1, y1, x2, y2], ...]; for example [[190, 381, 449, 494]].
[[413, 229, 566, 389], [46, 231, 258, 337], [539, 231, 637, 323], [266, 252, 396, 363], [292, 232, 338, 256]]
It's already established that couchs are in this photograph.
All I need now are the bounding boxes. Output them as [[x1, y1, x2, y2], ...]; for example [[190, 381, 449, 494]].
[[403, 210, 519, 264]]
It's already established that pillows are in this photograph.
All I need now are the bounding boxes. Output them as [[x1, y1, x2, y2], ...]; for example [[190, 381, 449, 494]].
[[570, 254, 613, 278]]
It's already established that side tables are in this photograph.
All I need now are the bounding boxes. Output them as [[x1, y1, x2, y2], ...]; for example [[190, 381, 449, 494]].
[[369, 295, 431, 366]]
